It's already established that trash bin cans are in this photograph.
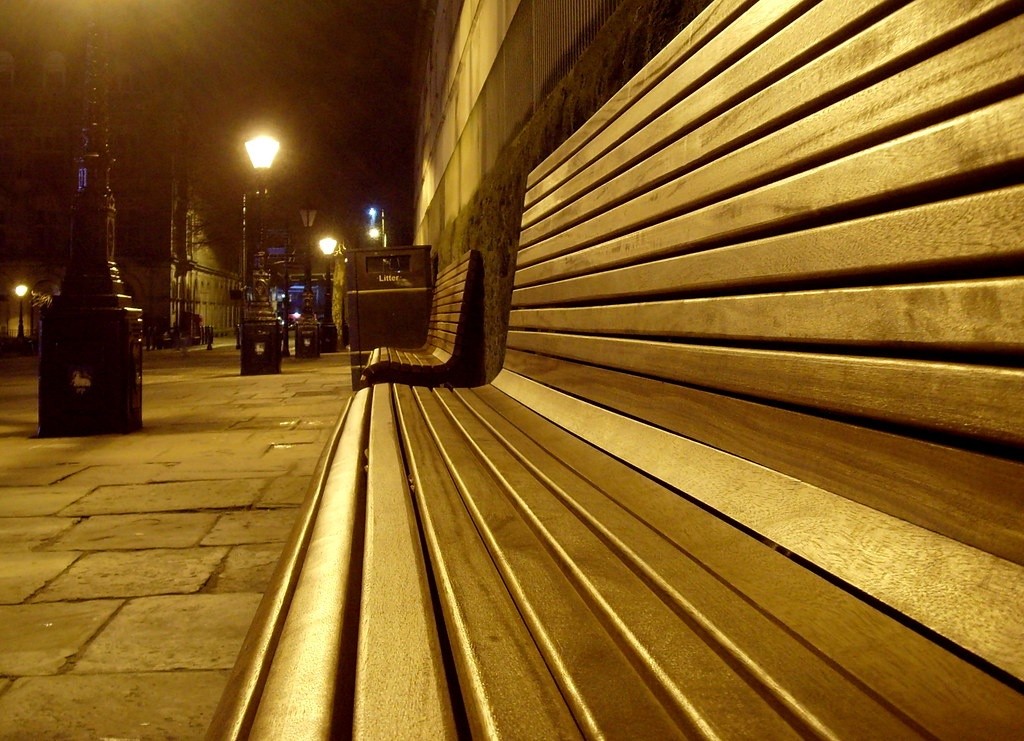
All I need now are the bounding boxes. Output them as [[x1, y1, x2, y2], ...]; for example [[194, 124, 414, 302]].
[[202, 325, 214, 345]]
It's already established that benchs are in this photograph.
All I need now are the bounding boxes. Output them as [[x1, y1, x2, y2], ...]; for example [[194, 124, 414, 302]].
[[359, 246, 477, 388], [204, 0, 1024, 740]]
